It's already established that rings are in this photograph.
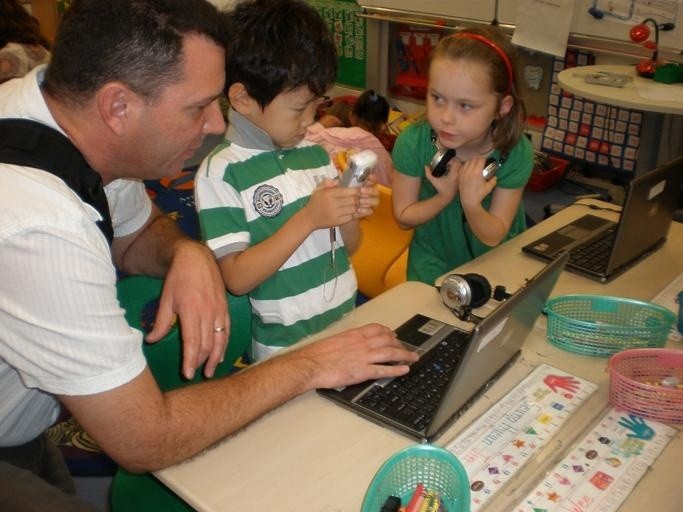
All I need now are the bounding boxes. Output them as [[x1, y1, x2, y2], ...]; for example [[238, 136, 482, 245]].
[[211, 327, 226, 332]]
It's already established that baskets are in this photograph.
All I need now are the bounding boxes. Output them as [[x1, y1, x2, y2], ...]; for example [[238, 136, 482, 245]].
[[543, 295, 677, 362], [359, 445, 473, 512], [608, 349, 683, 429]]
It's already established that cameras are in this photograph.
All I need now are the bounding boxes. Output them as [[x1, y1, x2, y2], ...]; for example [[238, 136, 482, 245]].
[[336, 149, 378, 191]]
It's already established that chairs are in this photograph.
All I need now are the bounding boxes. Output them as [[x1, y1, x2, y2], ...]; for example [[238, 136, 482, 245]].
[[335, 153, 415, 301], [108, 276, 253, 512]]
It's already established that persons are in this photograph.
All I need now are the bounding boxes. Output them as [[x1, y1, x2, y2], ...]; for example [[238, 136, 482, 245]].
[[390, 23, 534, 286], [317, 90, 388, 132], [0, 0, 419, 512], [193, 1, 379, 365], [0, 0, 51, 83]]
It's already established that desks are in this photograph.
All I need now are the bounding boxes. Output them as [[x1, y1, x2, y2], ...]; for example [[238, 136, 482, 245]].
[[556, 64, 683, 207], [435, 199, 683, 324], [151, 280, 682, 512]]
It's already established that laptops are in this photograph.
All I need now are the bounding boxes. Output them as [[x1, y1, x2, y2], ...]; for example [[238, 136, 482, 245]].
[[523, 155, 683, 285], [315, 251, 573, 444]]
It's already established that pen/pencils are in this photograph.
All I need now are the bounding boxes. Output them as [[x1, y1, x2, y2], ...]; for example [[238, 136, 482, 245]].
[[551, 317, 655, 355], [397, 483, 444, 512]]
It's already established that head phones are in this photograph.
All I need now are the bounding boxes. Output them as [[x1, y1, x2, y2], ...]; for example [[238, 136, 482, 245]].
[[440, 270, 515, 324], [428, 121, 507, 180]]
